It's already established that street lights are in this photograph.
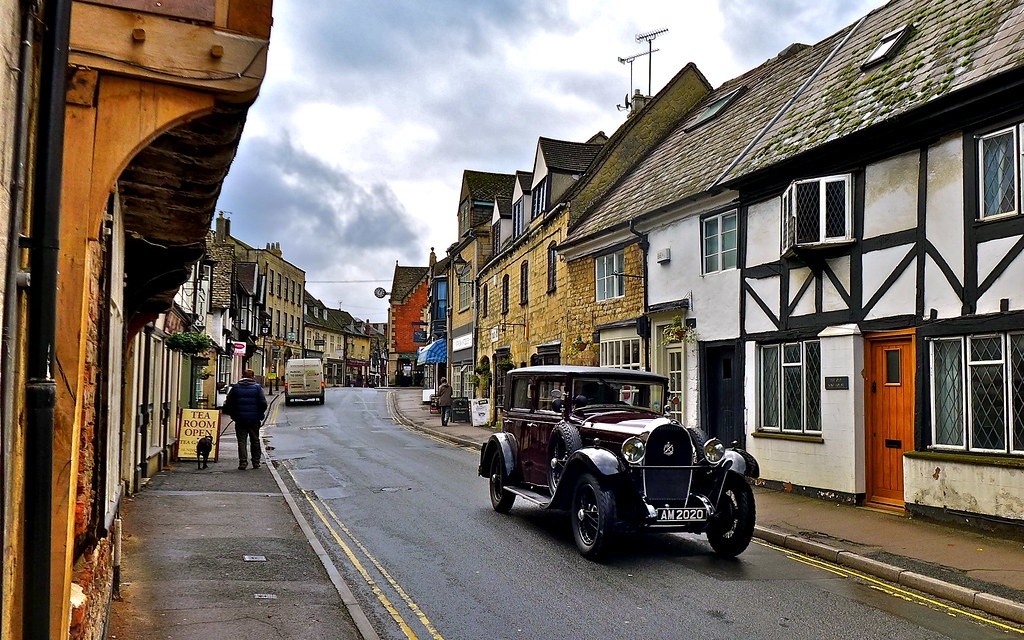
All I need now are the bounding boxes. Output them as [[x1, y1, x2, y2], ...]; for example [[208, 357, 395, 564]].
[[261, 323, 268, 379]]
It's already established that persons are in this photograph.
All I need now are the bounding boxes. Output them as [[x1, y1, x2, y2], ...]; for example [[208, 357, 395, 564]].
[[438, 377, 453, 427], [226, 369, 267, 470], [606, 389, 628, 405]]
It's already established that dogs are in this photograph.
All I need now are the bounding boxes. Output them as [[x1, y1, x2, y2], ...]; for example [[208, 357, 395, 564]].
[[197, 435, 214, 469]]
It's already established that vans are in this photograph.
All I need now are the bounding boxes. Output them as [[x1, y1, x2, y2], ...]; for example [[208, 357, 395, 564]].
[[282, 359, 326, 405]]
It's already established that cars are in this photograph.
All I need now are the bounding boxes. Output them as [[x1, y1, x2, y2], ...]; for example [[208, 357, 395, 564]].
[[477, 365, 762, 559]]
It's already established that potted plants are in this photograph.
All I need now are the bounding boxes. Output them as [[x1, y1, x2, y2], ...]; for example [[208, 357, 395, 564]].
[[656, 315, 701, 348]]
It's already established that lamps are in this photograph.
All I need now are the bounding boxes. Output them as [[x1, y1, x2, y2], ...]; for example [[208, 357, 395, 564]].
[[453, 252, 474, 297]]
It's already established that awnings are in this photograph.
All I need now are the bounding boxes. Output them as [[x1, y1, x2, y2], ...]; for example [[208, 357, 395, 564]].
[[417, 338, 446, 366]]
